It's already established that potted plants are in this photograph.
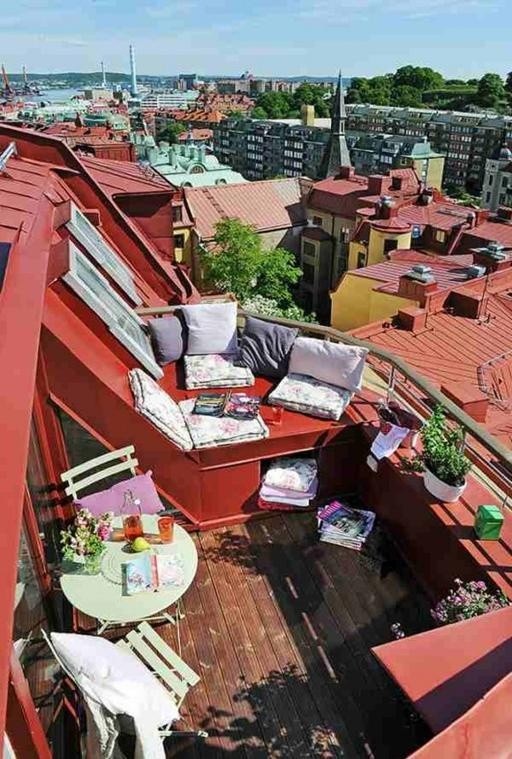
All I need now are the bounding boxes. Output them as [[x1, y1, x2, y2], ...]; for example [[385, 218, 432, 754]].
[[400, 402, 480, 503]]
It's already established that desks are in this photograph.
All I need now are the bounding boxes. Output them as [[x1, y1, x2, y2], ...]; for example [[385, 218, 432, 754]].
[[58, 514, 198, 635]]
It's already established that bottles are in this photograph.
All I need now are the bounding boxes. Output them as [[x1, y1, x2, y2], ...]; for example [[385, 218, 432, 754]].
[[119, 489, 143, 543]]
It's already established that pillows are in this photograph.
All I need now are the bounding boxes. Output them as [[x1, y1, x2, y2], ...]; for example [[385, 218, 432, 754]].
[[289, 337, 370, 393], [234, 314, 299, 380], [180, 303, 241, 355], [72, 470, 165, 515], [146, 315, 189, 366], [49, 631, 181, 728]]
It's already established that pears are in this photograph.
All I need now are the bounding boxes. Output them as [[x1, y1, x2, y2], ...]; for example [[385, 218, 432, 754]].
[[131, 536, 151, 553]]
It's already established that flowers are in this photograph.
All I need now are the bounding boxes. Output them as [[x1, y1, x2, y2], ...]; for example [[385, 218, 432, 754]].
[[389, 578, 510, 640]]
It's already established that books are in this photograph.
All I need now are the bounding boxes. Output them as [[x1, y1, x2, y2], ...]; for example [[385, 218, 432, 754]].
[[315, 500, 376, 552], [191, 388, 263, 421], [125, 551, 186, 596]]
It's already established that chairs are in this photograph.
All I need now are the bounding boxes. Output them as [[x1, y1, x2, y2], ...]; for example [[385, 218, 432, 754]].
[[39, 621, 209, 759], [59, 445, 158, 516]]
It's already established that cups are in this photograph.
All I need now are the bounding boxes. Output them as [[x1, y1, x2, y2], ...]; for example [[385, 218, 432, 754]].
[[156, 512, 175, 544]]
